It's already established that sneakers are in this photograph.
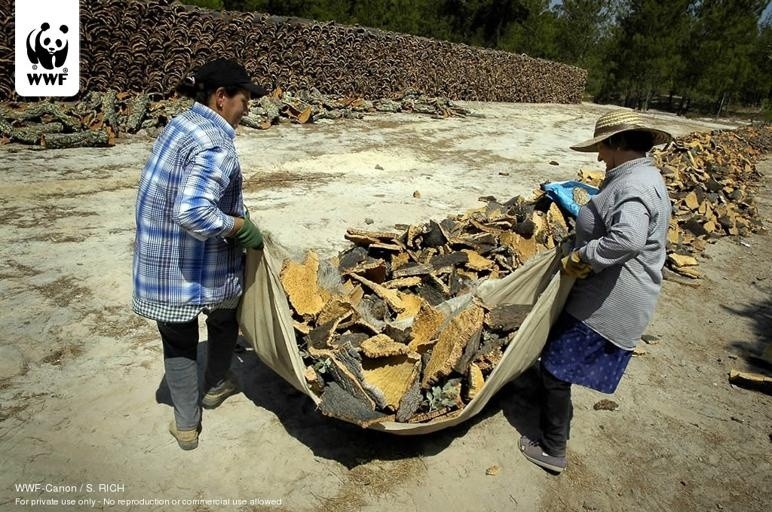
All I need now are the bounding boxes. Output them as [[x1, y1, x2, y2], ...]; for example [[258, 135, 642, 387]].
[[202, 369, 239, 410], [168, 418, 199, 451], [519, 433, 568, 473]]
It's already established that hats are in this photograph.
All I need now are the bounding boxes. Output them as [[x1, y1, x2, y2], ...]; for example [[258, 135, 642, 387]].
[[569, 108, 674, 154], [193, 60, 268, 101]]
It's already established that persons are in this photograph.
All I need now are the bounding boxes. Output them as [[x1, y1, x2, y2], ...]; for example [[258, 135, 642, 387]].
[[125, 57, 266, 451], [515, 107, 679, 474]]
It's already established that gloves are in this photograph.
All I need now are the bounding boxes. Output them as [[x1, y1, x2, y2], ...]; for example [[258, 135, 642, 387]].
[[559, 248, 590, 281], [233, 216, 266, 252]]
